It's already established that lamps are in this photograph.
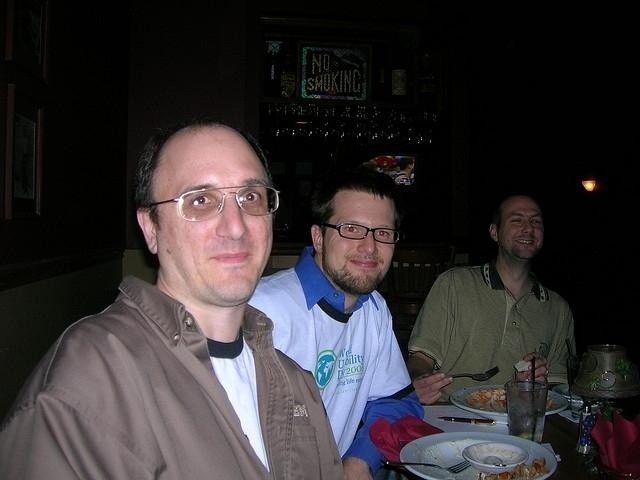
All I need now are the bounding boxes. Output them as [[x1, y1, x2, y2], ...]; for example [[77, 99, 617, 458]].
[[569, 342, 640, 477]]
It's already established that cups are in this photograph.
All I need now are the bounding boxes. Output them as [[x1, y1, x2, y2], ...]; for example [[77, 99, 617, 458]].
[[505, 379, 550, 443], [567, 357, 602, 419]]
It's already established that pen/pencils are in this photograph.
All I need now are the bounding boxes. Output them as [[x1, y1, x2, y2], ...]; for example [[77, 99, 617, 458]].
[[437, 417, 496, 426]]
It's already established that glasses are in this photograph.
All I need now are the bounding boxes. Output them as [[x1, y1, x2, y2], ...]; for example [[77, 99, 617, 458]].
[[322, 222, 400, 244], [148, 185, 281, 223]]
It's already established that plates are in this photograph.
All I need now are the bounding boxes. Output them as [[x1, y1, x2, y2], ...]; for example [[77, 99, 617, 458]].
[[553, 383, 583, 401], [450, 384, 569, 422], [399, 431, 557, 480], [461, 442, 529, 474]]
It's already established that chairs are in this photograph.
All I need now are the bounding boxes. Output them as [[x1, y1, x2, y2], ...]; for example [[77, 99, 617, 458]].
[[381, 239, 457, 367]]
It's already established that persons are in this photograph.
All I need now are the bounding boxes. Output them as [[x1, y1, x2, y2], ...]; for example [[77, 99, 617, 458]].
[[0, 118, 343, 480], [251, 163, 424, 480], [374, 154, 415, 187], [406, 188, 577, 404]]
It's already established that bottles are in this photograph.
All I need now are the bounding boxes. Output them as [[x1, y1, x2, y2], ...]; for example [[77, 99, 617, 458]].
[[577, 403, 596, 455]]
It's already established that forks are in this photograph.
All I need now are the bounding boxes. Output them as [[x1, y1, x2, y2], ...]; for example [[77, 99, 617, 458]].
[[423, 365, 501, 382], [382, 460, 472, 474]]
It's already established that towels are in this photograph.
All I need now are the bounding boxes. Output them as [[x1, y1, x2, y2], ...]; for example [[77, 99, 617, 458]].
[[587, 406, 640, 479], [369, 412, 445, 471]]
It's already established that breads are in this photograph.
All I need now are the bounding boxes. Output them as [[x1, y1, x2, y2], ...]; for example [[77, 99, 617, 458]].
[[466, 388, 490, 408], [490, 390, 507, 412]]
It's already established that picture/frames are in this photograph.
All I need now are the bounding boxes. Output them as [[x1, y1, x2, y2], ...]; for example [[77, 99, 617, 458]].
[[3, 82, 46, 221]]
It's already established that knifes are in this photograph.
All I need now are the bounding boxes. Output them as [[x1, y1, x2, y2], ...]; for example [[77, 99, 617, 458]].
[[437, 416, 496, 426]]
